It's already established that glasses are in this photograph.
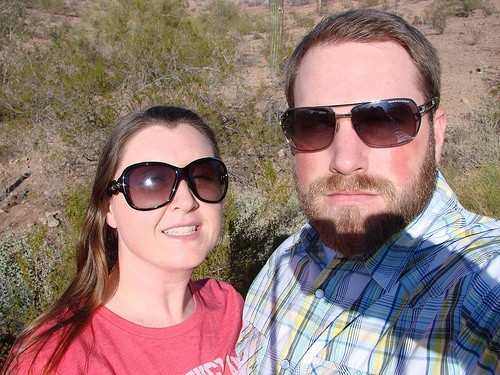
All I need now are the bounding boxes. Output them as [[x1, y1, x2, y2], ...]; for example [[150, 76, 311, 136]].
[[280, 98, 436, 152], [106, 157, 229, 211]]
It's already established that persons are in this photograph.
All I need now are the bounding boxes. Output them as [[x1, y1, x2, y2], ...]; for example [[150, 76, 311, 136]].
[[0, 104, 244, 375], [235, 12, 500, 375]]
